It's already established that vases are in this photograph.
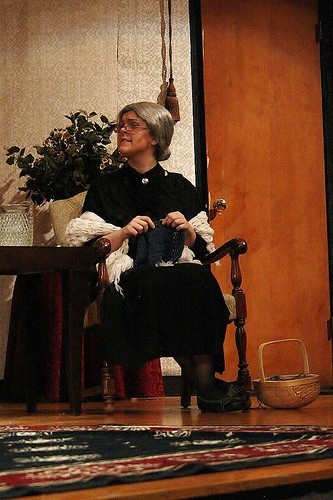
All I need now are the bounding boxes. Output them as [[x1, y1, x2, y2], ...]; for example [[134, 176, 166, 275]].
[[50, 190, 87, 246]]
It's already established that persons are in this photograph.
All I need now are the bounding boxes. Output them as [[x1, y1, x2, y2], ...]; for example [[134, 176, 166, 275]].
[[76, 102, 251, 412]]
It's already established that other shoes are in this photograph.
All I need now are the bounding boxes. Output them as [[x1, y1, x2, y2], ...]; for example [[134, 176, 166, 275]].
[[197, 392, 244, 410], [214, 379, 251, 409]]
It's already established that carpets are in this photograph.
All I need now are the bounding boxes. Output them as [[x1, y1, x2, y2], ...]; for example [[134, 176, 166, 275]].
[[0, 424, 333, 500]]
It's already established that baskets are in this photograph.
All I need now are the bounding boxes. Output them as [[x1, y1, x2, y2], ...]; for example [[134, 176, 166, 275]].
[[0, 202, 34, 246], [253, 339, 321, 409]]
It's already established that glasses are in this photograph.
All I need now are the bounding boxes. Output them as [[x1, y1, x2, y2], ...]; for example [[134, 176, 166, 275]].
[[113, 122, 149, 132]]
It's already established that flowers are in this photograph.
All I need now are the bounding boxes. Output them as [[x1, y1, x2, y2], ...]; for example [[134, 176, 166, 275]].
[[1, 108, 127, 206]]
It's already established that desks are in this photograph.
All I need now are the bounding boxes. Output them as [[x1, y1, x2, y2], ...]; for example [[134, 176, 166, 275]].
[[0, 245, 92, 416]]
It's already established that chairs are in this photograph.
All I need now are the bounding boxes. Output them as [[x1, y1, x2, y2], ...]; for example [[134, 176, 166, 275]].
[[93, 237, 251, 416]]
